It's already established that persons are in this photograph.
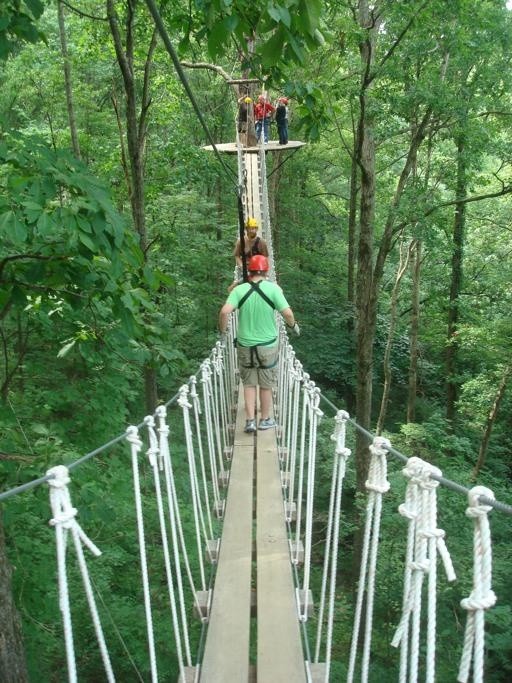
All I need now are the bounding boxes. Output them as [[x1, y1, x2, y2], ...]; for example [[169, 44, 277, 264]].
[[234, 93, 252, 147], [275, 96, 289, 145], [233, 217, 268, 273], [252, 95, 276, 145], [218, 252, 303, 432], [226, 269, 274, 292]]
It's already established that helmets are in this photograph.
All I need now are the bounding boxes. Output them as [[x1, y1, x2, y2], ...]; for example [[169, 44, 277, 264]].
[[245, 218, 258, 228], [248, 254, 270, 272], [244, 97, 252, 104]]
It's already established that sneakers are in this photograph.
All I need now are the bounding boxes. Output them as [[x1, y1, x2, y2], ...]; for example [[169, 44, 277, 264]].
[[257, 417, 277, 430], [243, 417, 256, 432]]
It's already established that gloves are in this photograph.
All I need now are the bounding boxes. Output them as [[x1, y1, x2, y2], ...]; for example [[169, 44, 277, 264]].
[[287, 323, 300, 337]]
